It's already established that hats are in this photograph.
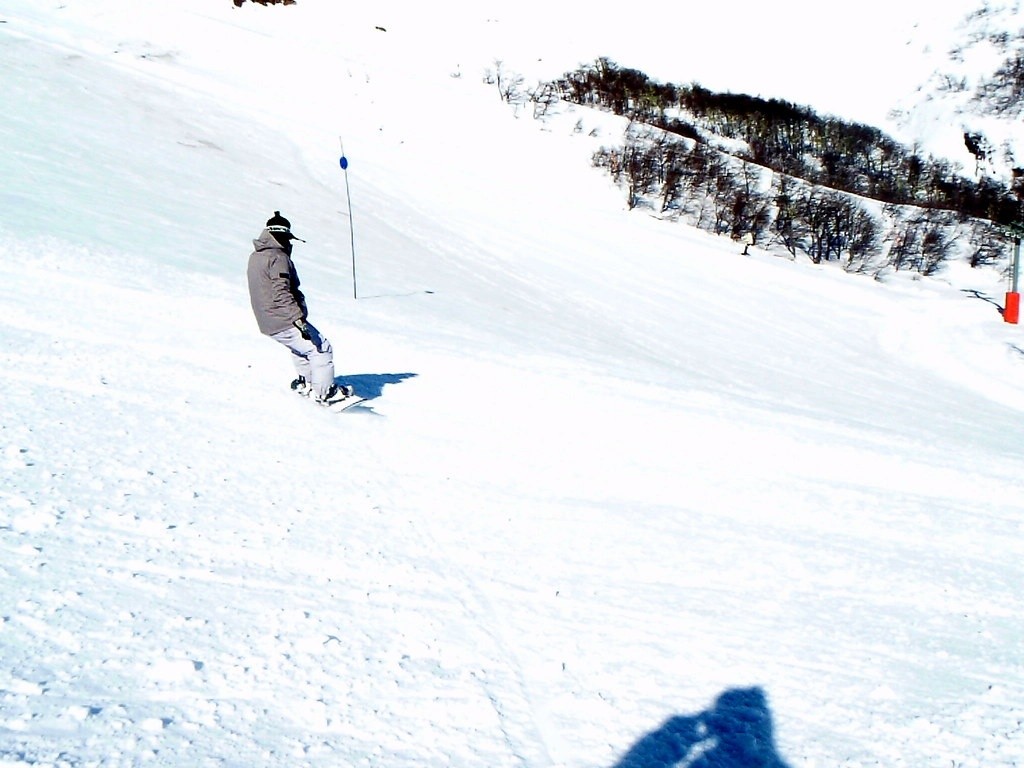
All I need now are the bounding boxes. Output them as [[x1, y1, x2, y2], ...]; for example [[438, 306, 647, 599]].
[[267, 212, 306, 242]]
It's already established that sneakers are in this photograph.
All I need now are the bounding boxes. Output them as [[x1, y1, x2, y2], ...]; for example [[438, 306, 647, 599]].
[[314, 382, 354, 407], [292, 373, 313, 395]]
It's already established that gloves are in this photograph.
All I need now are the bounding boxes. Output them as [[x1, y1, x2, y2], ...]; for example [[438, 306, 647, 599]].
[[292, 318, 312, 340]]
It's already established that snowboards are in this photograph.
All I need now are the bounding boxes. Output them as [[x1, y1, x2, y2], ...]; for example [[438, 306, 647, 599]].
[[290, 377, 368, 413]]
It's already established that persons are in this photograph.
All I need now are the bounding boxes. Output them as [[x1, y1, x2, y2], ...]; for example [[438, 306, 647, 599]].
[[248, 211, 353, 403]]
